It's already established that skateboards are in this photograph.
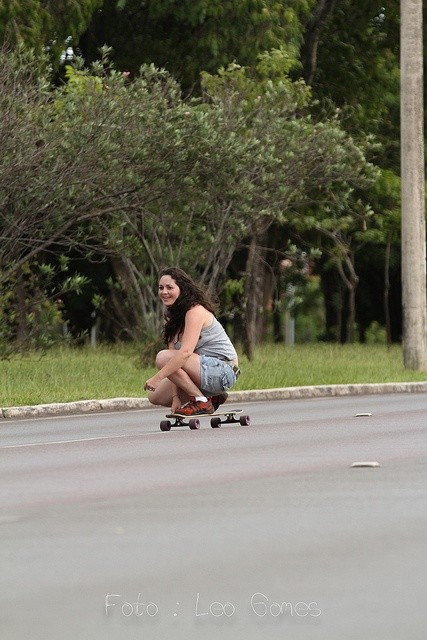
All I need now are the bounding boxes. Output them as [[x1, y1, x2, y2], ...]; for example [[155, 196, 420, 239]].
[[160, 409, 250, 431]]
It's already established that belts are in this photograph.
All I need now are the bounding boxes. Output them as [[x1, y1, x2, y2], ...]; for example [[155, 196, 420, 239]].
[[216, 354, 241, 381]]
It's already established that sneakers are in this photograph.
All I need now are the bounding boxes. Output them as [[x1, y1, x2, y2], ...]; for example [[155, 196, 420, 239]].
[[175, 396, 215, 416], [211, 393, 228, 411]]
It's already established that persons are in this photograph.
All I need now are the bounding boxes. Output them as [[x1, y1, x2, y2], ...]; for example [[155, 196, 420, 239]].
[[142, 267, 244, 420]]
